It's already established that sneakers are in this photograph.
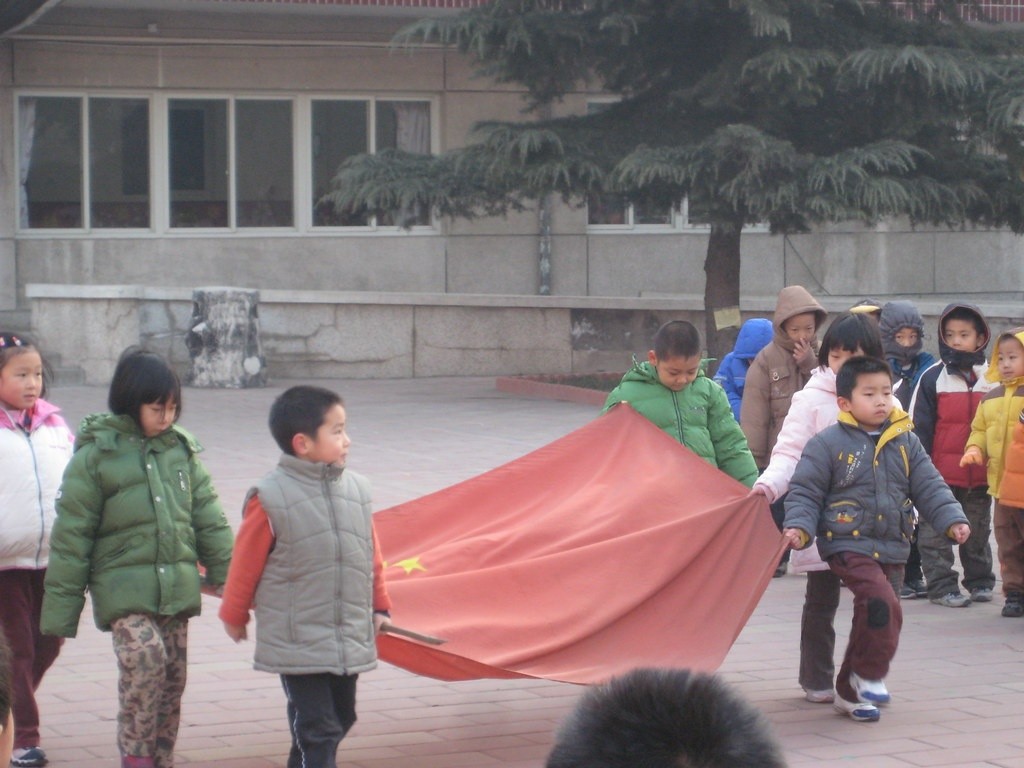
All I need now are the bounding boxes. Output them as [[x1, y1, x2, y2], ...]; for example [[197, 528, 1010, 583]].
[[971, 587, 993, 601], [832, 693, 880, 722], [10, 746, 49, 767], [1002, 591, 1024, 617], [849, 672, 891, 704], [900, 579, 928, 599], [932, 593, 971, 607], [802, 685, 835, 702]]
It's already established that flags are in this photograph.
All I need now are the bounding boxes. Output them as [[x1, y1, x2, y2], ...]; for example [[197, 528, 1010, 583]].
[[198, 402, 791, 687]]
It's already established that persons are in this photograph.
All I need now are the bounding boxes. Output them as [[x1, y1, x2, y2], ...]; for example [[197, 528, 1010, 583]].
[[544, 664, 785, 768], [219, 386, 393, 768], [846, 299, 997, 608], [750, 313, 888, 703], [601, 321, 760, 490], [0, 332, 76, 768], [959, 327, 1024, 618], [783, 356, 971, 722], [713, 285, 827, 579], [40, 345, 235, 768]]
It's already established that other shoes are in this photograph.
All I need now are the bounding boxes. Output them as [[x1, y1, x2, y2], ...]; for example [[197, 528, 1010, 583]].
[[773, 562, 787, 578], [122, 753, 158, 768]]
